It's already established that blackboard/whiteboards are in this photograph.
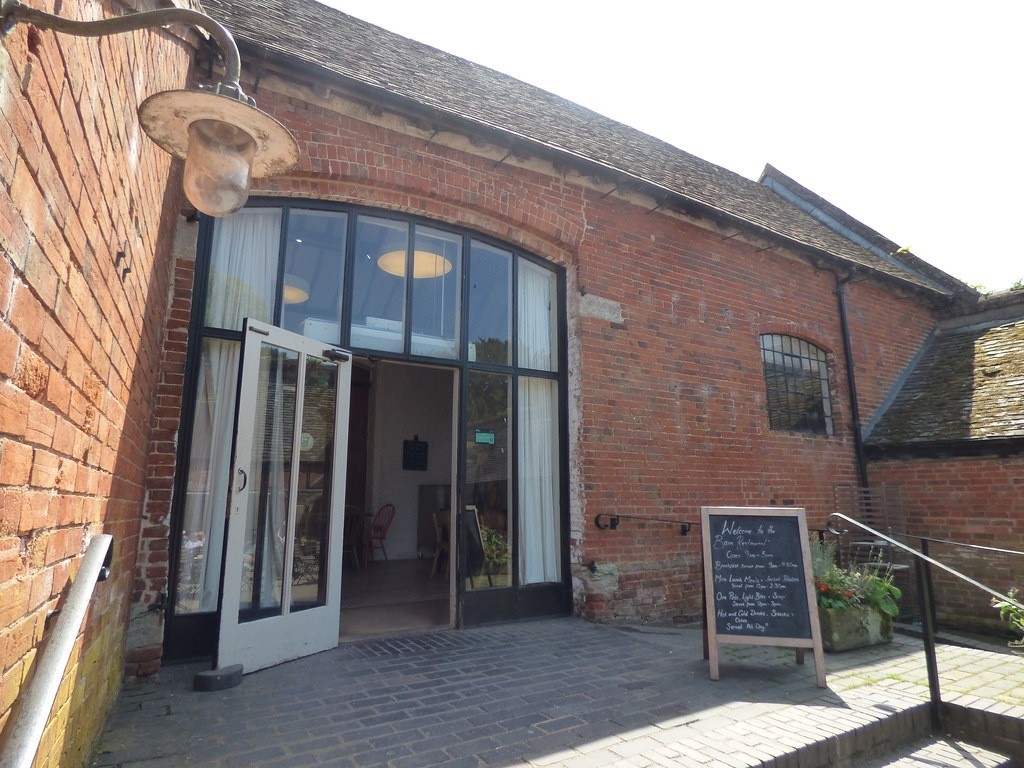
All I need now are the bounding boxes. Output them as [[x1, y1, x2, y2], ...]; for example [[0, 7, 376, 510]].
[[699, 504, 823, 648]]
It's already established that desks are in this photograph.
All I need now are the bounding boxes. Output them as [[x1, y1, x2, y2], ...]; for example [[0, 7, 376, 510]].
[[345, 511, 372, 536]]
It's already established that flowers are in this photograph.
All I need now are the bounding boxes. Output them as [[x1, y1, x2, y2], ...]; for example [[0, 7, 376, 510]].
[[810, 533, 902, 617]]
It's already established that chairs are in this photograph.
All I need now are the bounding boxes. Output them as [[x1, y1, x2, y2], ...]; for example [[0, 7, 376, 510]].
[[344, 504, 365, 572], [293, 542, 320, 585], [431, 511, 451, 582], [355, 504, 396, 569]]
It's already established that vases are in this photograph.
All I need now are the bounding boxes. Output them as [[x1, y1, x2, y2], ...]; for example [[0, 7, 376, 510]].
[[816, 603, 893, 653]]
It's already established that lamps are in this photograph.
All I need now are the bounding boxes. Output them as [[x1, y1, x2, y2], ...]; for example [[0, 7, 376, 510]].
[[0, 0, 302, 217]]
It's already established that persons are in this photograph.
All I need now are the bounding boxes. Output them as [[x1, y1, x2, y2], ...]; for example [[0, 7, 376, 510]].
[[181, 529, 206, 582]]
